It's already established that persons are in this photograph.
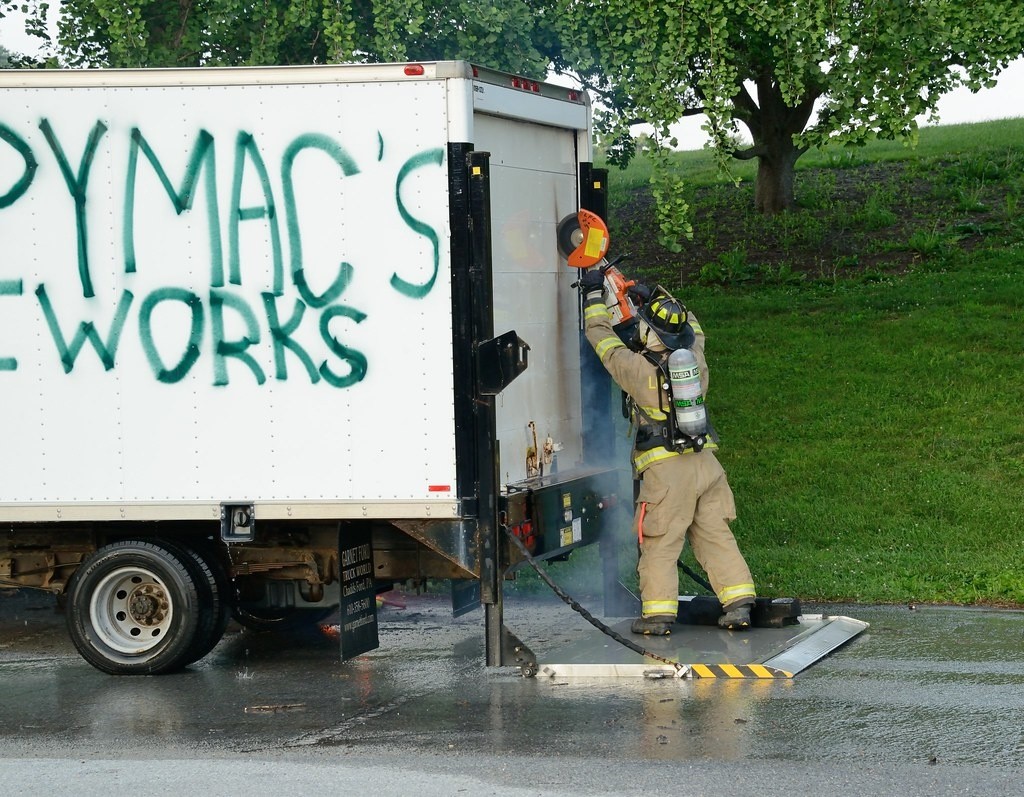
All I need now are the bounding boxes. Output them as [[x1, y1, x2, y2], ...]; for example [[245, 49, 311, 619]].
[[580, 267, 755, 635]]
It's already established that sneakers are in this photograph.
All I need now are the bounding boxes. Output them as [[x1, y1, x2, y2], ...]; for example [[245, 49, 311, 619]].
[[631, 618, 672, 635], [718, 604, 751, 630]]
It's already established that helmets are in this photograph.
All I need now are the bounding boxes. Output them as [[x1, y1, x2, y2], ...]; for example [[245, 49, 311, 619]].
[[638, 295, 696, 351]]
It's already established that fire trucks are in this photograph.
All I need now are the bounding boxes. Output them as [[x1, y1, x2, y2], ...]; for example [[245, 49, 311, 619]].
[[0, 62, 622, 677]]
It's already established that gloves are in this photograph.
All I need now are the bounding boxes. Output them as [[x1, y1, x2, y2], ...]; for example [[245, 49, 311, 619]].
[[580, 266, 605, 295], [629, 284, 650, 303]]
